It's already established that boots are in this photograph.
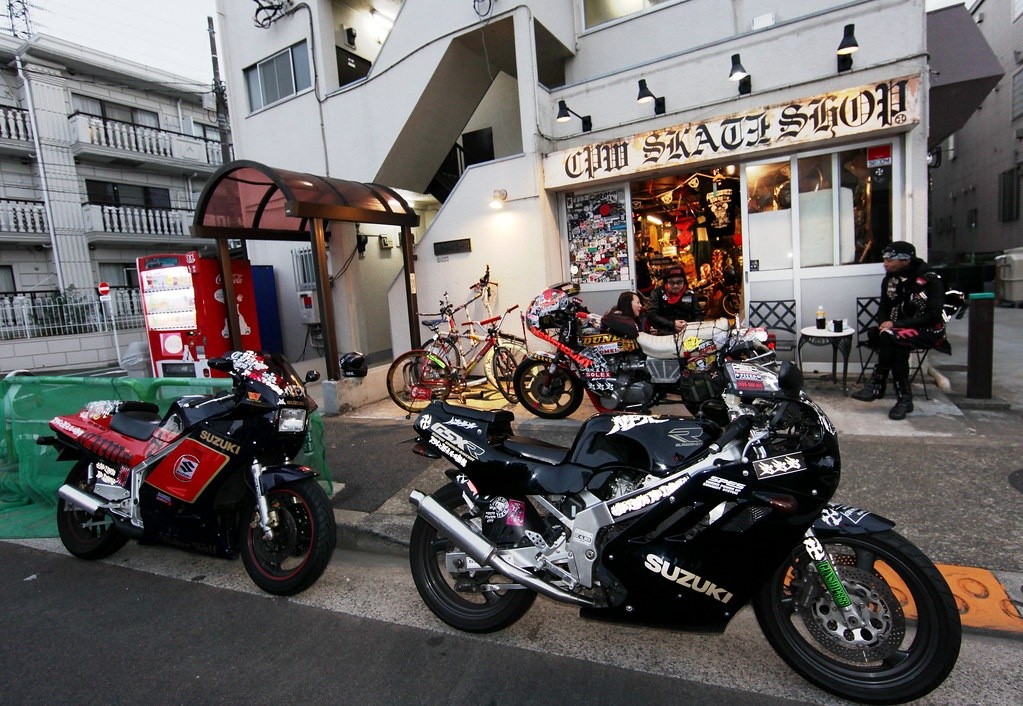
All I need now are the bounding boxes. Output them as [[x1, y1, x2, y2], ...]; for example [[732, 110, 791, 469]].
[[852, 365, 890, 402], [890, 376, 913, 419]]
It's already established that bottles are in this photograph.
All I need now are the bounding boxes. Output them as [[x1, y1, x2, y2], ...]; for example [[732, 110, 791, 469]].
[[815, 305, 826, 329]]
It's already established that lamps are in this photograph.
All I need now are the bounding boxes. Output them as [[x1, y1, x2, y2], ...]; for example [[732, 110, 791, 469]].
[[835, 23, 859, 72], [729, 54, 752, 94], [557, 100, 591, 131], [637, 79, 665, 116]]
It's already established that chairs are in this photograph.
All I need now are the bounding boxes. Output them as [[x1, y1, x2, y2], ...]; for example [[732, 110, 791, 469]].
[[854, 296, 929, 402], [748, 299, 796, 366]]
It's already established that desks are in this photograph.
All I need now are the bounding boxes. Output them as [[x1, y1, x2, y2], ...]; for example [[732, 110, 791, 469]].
[[796, 325, 855, 396]]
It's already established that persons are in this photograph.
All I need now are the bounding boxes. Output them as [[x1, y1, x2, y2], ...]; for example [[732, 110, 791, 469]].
[[599, 292, 642, 339], [642, 266, 702, 336], [851, 241, 945, 420]]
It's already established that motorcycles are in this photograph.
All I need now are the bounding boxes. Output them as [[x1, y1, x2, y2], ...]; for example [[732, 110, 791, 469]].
[[409, 334, 965, 706], [37, 346, 337, 598], [513, 260, 778, 420]]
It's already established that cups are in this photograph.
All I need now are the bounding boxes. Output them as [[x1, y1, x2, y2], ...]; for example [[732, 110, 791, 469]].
[[833, 319, 843, 332]]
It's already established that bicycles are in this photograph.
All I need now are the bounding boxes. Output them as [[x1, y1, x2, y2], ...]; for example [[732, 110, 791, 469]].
[[386, 266, 543, 420]]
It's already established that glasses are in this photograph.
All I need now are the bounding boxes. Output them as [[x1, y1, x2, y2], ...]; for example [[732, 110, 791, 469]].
[[667, 279, 684, 286]]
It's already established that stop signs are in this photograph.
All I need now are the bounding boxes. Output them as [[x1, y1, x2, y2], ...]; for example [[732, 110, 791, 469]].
[[98, 282, 109, 295]]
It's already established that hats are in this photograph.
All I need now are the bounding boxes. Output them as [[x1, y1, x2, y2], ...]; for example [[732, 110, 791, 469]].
[[665, 267, 684, 277], [881, 241, 915, 259]]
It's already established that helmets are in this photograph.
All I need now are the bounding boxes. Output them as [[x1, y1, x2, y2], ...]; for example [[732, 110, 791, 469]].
[[340, 351, 368, 377]]
